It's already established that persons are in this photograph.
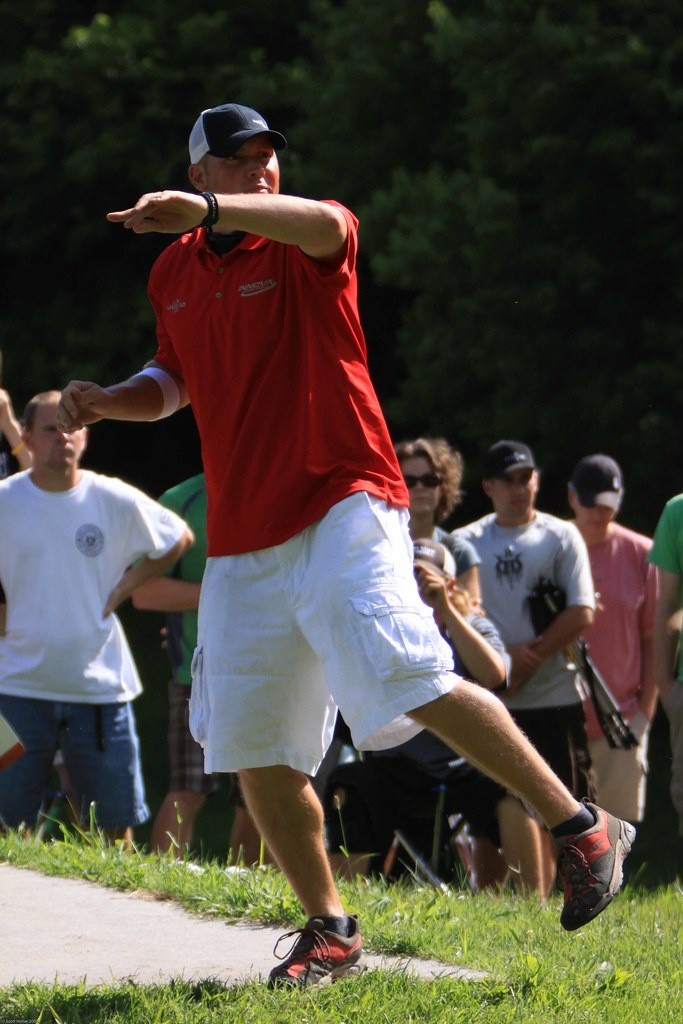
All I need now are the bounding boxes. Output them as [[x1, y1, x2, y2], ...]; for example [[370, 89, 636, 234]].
[[0, 388, 683, 903], [61, 103, 636, 992]]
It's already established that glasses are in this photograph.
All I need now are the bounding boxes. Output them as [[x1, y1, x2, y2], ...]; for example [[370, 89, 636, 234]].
[[402, 472, 440, 489]]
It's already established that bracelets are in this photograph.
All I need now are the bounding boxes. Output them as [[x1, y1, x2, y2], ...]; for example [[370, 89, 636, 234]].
[[11, 442, 23, 456], [200, 191, 219, 243]]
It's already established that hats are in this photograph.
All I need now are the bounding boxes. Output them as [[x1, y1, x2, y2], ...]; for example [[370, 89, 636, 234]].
[[414, 538, 455, 585], [189, 103, 287, 164], [483, 440, 535, 478], [570, 454, 621, 511]]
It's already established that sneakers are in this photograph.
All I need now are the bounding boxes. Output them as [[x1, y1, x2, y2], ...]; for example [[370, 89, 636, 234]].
[[266, 914, 361, 994], [552, 796, 636, 931]]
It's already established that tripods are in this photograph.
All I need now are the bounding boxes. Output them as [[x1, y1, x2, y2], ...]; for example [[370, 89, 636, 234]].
[[526, 575, 641, 750]]
